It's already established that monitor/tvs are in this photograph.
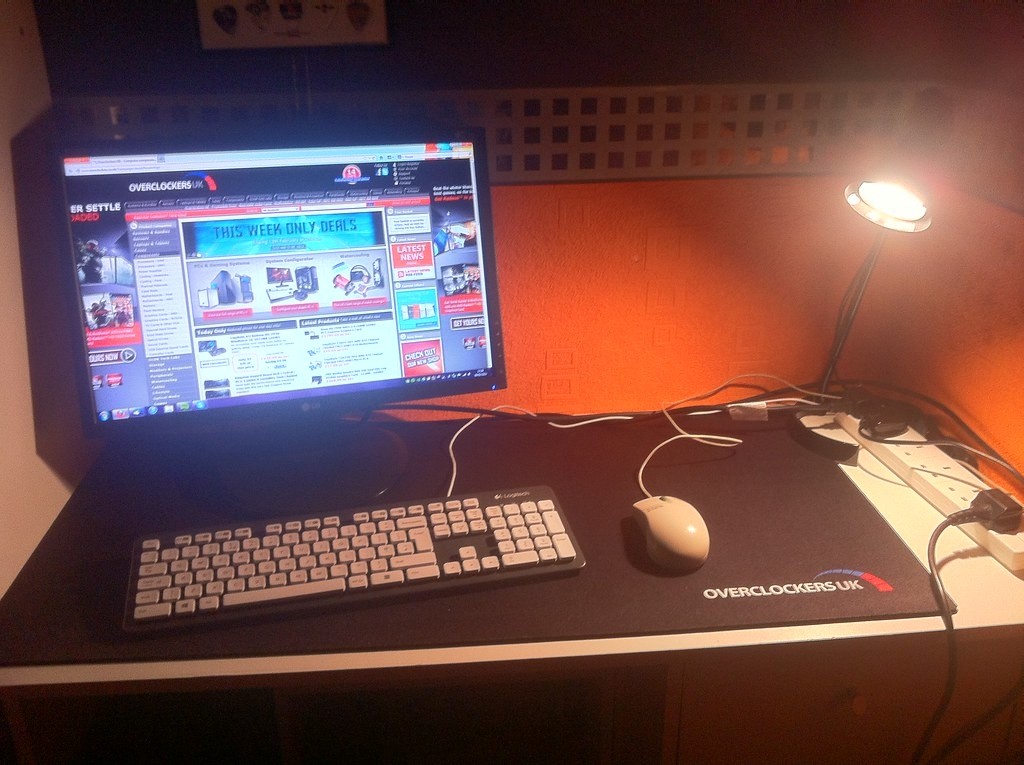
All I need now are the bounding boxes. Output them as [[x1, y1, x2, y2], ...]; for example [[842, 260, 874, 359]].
[[46, 125, 507, 511]]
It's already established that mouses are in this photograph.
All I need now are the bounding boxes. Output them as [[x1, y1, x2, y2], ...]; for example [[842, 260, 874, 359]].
[[631, 493, 709, 571]]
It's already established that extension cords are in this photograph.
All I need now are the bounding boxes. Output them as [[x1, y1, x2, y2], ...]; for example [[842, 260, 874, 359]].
[[835, 410, 1024, 572]]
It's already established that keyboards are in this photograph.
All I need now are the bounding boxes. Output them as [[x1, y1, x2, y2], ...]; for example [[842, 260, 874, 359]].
[[123, 488, 587, 634]]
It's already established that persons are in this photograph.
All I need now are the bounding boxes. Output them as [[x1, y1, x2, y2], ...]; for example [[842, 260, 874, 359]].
[[79, 238, 104, 285], [90, 299, 132, 326]]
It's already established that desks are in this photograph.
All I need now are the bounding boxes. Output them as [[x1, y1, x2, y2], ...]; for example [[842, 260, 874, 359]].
[[0, 403, 1024, 764]]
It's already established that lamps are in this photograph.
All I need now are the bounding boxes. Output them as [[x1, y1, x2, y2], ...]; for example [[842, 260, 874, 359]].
[[790, 88, 949, 462]]
